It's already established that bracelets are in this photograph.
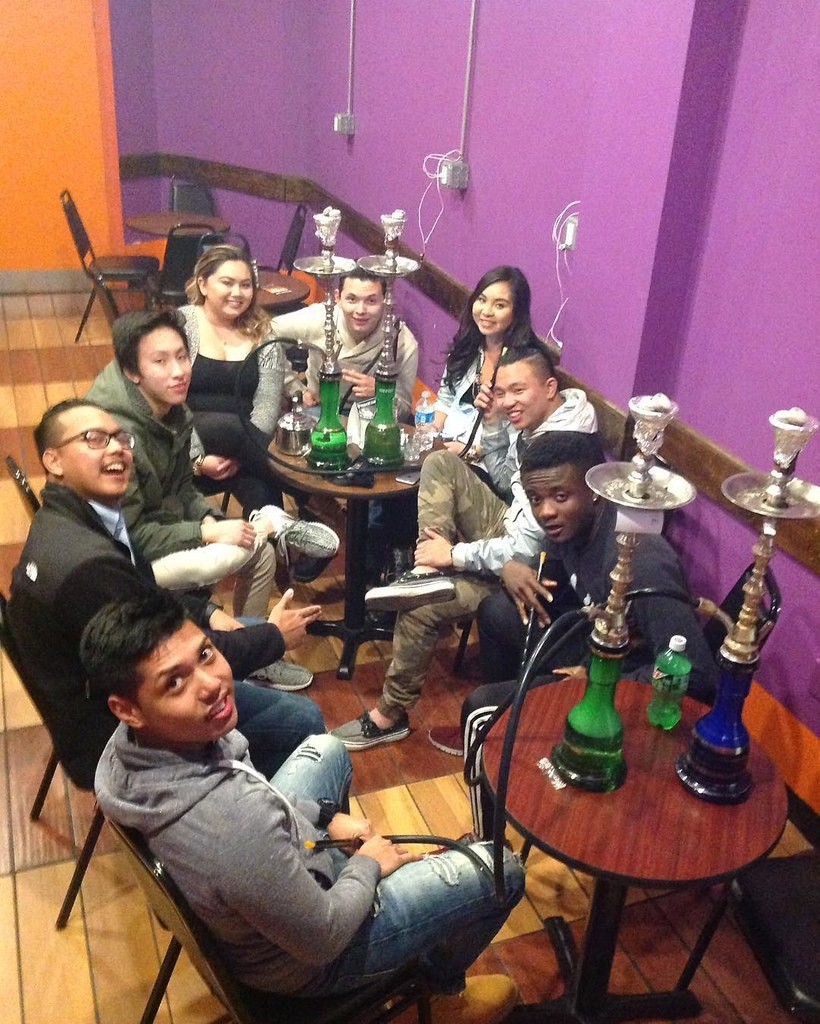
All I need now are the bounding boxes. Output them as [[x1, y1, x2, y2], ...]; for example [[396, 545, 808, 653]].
[[193, 454, 204, 476]]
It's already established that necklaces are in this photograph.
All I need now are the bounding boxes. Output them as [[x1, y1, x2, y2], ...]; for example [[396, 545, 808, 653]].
[[475, 375, 481, 394]]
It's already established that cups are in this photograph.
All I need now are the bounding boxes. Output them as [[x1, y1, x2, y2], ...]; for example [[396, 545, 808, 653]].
[[404, 436, 421, 460]]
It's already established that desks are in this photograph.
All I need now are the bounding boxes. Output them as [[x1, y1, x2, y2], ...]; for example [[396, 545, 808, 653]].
[[264, 413, 447, 681], [120, 213, 230, 237], [481, 680, 789, 1024], [186, 269, 310, 309]]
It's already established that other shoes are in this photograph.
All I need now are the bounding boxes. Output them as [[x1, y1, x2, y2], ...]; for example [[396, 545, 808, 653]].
[[295, 551, 338, 583]]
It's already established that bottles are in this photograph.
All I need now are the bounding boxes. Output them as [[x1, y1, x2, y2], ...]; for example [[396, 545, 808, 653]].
[[646, 635, 692, 730], [414, 391, 435, 451]]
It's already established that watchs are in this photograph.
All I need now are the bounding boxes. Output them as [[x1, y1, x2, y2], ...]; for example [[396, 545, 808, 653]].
[[317, 798, 340, 830]]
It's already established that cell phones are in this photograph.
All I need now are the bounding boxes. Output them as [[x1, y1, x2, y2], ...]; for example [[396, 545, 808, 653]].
[[395, 471, 420, 486]]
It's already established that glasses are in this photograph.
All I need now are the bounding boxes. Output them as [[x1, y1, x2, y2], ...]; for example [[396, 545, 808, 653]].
[[53, 427, 136, 451]]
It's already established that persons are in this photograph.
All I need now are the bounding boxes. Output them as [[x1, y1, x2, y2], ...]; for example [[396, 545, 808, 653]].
[[5, 398, 322, 776], [427, 430, 718, 857], [271, 258, 418, 501], [171, 243, 331, 597], [79, 586, 526, 1000], [364, 265, 546, 581], [78, 309, 340, 689], [327, 344, 599, 751]]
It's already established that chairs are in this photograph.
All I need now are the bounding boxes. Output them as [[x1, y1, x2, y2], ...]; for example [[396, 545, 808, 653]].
[[104, 816, 435, 1024], [674, 854, 820, 1024], [58, 171, 307, 343], [517, 562, 782, 865], [5, 455, 40, 521], [0, 589, 106, 929], [451, 494, 608, 675]]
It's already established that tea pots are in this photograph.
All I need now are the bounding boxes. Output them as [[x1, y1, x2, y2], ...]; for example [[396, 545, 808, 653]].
[[276, 390, 316, 455]]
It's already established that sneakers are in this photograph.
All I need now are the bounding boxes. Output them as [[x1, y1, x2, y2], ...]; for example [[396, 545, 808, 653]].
[[257, 505, 341, 567], [387, 975, 519, 1024], [327, 710, 411, 751], [364, 569, 455, 611], [428, 724, 465, 756], [243, 662, 313, 691]]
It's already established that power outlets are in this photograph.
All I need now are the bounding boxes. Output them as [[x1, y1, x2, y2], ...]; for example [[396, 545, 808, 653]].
[[333, 113, 355, 135], [440, 162, 469, 189], [564, 217, 578, 250]]
